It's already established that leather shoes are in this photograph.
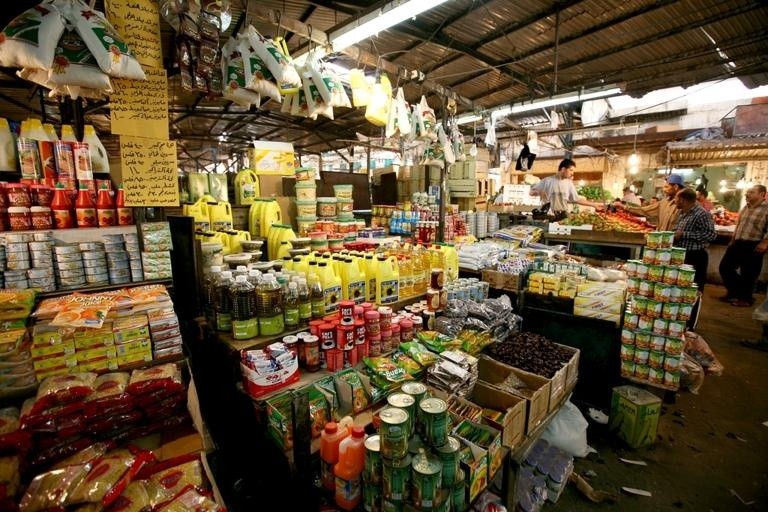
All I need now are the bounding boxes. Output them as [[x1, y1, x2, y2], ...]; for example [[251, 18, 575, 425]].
[[733, 300, 752, 307], [719, 294, 733, 301]]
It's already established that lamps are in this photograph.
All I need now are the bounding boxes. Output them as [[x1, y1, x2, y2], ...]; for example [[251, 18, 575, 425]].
[[491, 80, 627, 119], [328, 0, 460, 53], [436, 107, 484, 127], [286, 38, 330, 67]]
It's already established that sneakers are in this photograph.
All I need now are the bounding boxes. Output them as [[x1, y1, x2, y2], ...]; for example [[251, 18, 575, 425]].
[[740, 338, 767, 350]]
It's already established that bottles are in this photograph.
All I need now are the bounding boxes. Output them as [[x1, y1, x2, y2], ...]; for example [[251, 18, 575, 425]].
[[372, 221, 459, 300], [204, 264, 327, 341], [50, 183, 135, 230]]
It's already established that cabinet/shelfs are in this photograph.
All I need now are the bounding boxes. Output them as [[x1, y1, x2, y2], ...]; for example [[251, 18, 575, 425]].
[[523, 295, 623, 386], [197, 293, 430, 479]]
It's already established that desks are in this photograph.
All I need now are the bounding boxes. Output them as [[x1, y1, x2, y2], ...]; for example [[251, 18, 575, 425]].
[[542, 231, 648, 260]]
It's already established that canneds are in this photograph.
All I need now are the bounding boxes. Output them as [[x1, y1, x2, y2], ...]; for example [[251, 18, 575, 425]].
[[440, 277, 489, 302], [283, 331, 321, 372], [0, 182, 53, 232], [362, 382, 467, 512], [617, 231, 697, 390], [404, 300, 443, 330], [0, 234, 143, 292]]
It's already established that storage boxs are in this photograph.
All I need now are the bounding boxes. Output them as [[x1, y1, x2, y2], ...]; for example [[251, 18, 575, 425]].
[[397, 146, 488, 202], [248, 137, 296, 176], [448, 340, 582, 502], [611, 385, 661, 451]]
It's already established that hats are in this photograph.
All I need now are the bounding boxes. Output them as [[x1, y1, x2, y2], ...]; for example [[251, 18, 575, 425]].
[[667, 175, 685, 188]]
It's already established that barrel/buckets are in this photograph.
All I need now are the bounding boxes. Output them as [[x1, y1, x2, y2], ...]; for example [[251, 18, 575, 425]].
[[333, 425, 369, 510], [0, 117, 110, 172], [282, 249, 400, 315], [235, 169, 260, 205], [430, 242, 458, 284], [268, 225, 296, 262], [321, 416, 353, 489], [182, 191, 252, 256], [249, 197, 282, 238]]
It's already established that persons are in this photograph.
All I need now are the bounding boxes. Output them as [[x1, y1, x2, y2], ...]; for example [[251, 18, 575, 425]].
[[673, 188, 716, 329], [611, 176, 686, 235], [529, 159, 606, 254], [719, 184, 768, 307], [697, 188, 768, 224], [606, 186, 660, 217]]
